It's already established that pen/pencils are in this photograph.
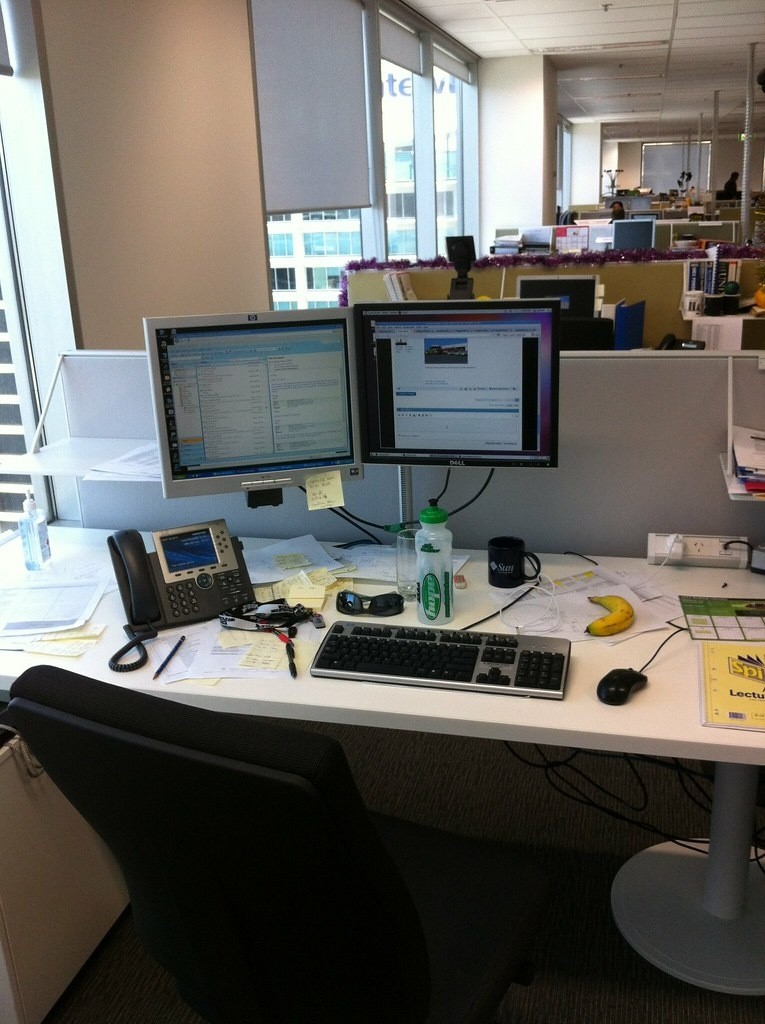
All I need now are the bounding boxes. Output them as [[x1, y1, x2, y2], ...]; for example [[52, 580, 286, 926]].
[[271, 627, 296, 647], [285, 643, 297, 681], [152, 635, 186, 680]]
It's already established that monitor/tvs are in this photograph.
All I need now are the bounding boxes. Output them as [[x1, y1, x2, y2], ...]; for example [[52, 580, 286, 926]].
[[629, 212, 659, 221], [611, 219, 655, 250], [143, 275, 598, 500]]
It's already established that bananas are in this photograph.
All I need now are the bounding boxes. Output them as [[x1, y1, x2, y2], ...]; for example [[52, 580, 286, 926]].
[[588, 596, 634, 636]]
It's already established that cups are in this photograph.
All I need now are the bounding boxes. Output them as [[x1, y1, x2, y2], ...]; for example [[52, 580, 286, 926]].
[[681, 290, 703, 317], [488, 536, 541, 588], [396, 528, 418, 602]]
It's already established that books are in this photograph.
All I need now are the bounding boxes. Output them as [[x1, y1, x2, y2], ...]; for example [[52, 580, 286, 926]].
[[383, 271, 418, 300], [697, 639, 765, 732]]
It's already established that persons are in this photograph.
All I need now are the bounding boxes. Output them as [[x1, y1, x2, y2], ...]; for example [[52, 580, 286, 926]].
[[724, 172, 739, 199], [732, 424, 765, 496], [684, 245, 742, 295]]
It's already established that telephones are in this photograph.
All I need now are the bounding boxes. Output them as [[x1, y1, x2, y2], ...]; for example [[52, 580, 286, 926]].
[[105, 517, 255, 673]]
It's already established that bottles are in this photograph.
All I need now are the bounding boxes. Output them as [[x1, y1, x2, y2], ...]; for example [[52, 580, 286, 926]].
[[18, 488, 52, 570], [414, 499, 454, 625]]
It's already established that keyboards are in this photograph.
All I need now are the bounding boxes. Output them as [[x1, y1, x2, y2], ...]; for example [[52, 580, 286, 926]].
[[311, 620, 571, 699]]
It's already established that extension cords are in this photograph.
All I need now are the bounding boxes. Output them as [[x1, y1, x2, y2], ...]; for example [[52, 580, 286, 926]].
[[647, 532, 748, 570]]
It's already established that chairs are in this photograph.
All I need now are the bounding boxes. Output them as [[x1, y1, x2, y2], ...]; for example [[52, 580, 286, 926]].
[[7, 665, 549, 1024]]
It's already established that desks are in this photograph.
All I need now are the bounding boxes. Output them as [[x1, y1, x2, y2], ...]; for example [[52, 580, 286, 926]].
[[0, 525, 765, 997]]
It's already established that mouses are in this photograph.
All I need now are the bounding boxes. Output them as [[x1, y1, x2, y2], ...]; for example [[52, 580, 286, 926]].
[[596, 668, 647, 706]]
